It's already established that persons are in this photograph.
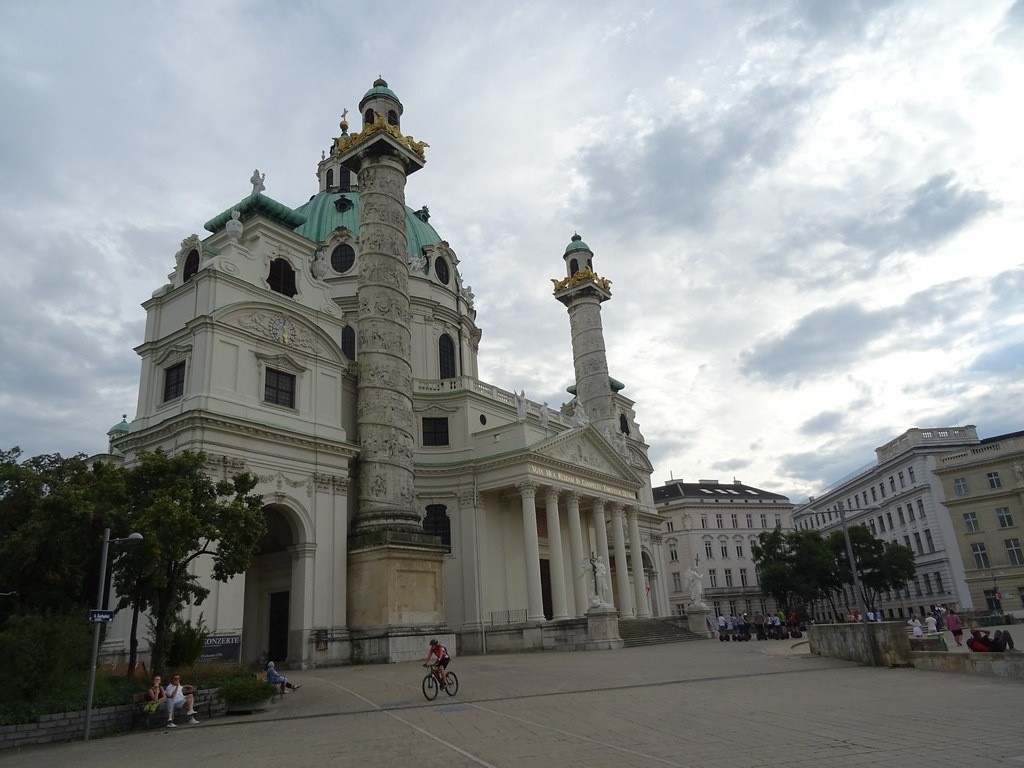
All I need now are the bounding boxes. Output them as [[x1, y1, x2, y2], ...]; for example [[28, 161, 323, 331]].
[[946, 609, 963, 646], [907, 614, 923, 638], [620, 432, 626, 449], [753, 610, 765, 636], [718, 614, 727, 638], [251, 170, 266, 192], [572, 397, 586, 418], [539, 402, 548, 426], [730, 613, 749, 638], [925, 604, 947, 634], [604, 426, 610, 440], [867, 609, 882, 622], [165, 674, 199, 725], [970, 628, 1019, 652], [789, 609, 800, 635], [267, 661, 301, 694], [765, 611, 787, 636], [1009, 462, 1023, 481], [424, 639, 449, 688], [514, 389, 527, 419], [148, 673, 178, 727], [854, 611, 859, 623], [811, 619, 816, 625]]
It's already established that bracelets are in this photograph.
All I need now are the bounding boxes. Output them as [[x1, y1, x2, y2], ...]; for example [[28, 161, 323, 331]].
[[438, 659, 441, 661]]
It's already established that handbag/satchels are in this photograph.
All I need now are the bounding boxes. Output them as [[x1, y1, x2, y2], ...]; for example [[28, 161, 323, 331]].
[[143, 701, 160, 713]]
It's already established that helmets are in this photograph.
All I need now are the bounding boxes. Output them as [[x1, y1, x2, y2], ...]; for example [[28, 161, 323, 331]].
[[430, 639, 438, 645]]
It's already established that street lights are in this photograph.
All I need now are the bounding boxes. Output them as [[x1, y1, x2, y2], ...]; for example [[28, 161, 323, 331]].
[[84, 532, 143, 740], [801, 504, 882, 667]]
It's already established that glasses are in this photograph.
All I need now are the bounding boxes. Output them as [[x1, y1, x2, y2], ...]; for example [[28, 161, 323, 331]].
[[174, 678, 180, 680]]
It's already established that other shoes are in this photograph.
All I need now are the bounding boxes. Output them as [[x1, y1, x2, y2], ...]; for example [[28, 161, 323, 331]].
[[444, 683, 449, 688], [187, 709, 197, 716], [168, 717, 173, 721], [283, 691, 290, 694], [292, 685, 301, 690], [188, 717, 199, 724], [167, 723, 178, 728], [1010, 648, 1020, 652]]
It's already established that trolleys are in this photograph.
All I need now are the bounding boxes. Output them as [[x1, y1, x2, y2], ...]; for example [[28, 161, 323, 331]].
[[719, 620, 802, 642]]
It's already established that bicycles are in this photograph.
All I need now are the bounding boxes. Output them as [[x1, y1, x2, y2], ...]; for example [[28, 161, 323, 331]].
[[421, 663, 458, 701]]
